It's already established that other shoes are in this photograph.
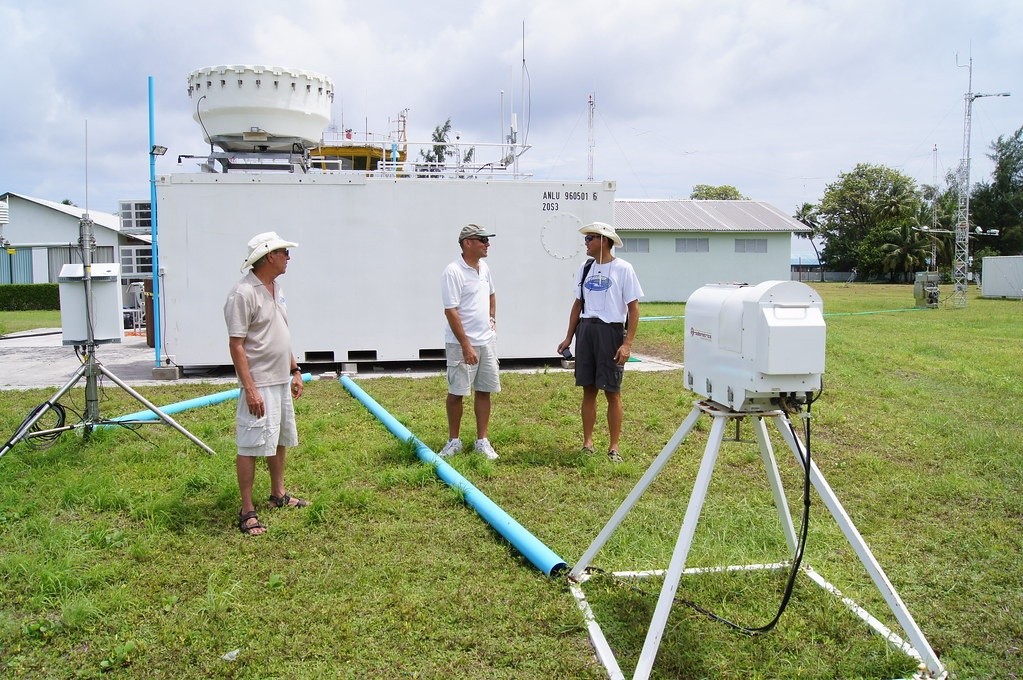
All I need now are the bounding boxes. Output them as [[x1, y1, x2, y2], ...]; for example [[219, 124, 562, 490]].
[[609, 449, 622, 463], [581, 446, 594, 454]]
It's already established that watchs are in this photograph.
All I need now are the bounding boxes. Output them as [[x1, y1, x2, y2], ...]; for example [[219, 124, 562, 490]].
[[291, 366, 302, 374]]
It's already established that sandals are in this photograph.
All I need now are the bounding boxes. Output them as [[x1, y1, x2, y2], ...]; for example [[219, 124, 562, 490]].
[[240, 508, 267, 537], [268, 492, 313, 509]]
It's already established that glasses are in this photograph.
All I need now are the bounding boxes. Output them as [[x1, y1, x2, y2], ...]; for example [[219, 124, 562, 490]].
[[585, 234, 606, 243], [273, 249, 289, 257], [465, 237, 489, 243]]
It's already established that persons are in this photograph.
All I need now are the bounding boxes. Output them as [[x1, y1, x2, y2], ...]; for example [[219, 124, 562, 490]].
[[224, 231, 314, 535], [558, 221, 645, 463], [438, 223, 502, 461]]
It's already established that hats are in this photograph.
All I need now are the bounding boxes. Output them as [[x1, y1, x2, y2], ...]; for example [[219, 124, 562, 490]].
[[241, 233, 299, 268], [458, 224, 496, 243], [579, 222, 623, 248]]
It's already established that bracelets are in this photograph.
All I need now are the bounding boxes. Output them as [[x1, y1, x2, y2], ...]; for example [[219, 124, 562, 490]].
[[490, 316, 496, 323]]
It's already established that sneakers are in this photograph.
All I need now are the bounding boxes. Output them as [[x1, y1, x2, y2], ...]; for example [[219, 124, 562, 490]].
[[438, 436, 463, 457], [475, 438, 499, 460]]
